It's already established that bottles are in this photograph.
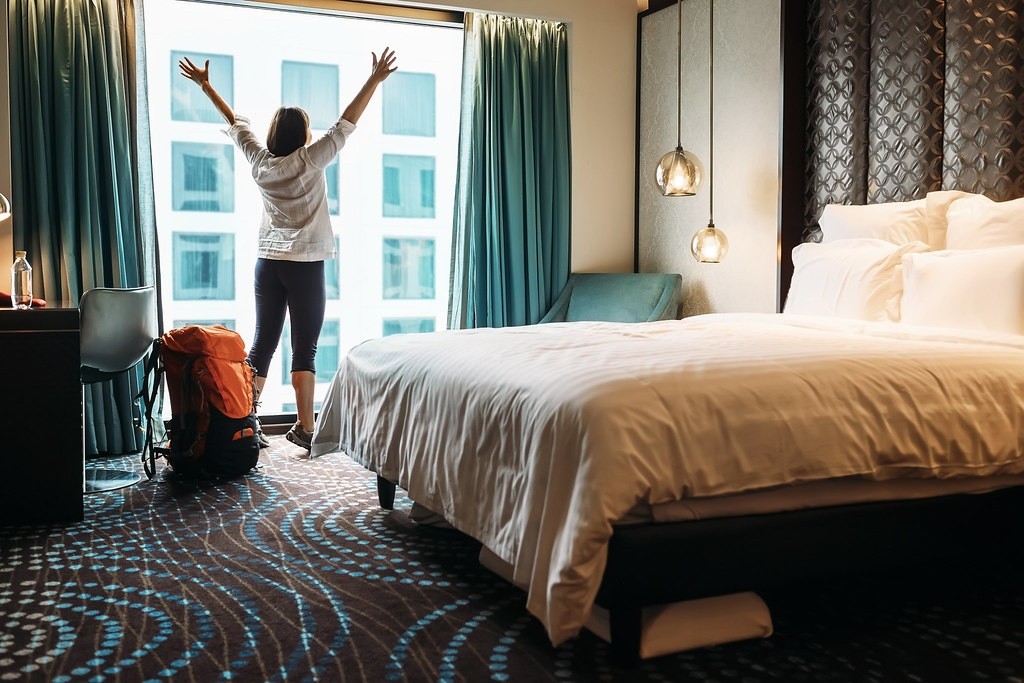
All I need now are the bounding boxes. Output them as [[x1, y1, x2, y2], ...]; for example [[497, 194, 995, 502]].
[[11, 251, 33, 310]]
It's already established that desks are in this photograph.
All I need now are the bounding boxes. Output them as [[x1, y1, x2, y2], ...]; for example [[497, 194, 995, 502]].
[[0, 299, 85, 530]]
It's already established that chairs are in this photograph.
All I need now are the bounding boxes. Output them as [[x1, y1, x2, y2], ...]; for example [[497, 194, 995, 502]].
[[526, 271, 683, 324], [79, 283, 162, 479]]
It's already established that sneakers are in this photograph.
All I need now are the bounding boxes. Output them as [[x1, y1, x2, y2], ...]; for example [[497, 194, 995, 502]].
[[287, 420, 315, 450], [255, 416, 270, 447]]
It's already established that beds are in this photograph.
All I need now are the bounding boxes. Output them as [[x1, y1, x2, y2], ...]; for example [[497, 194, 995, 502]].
[[307, 310, 1024, 681]]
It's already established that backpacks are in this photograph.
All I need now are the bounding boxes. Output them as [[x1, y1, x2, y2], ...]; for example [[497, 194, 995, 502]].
[[135, 324, 260, 482]]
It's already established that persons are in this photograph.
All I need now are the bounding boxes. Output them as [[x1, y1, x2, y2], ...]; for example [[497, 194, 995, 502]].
[[178, 47, 400, 453]]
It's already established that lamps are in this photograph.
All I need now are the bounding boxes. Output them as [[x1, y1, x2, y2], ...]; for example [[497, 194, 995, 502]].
[[0, 192, 13, 224], [696, 0, 732, 263], [660, 0, 700, 197]]
